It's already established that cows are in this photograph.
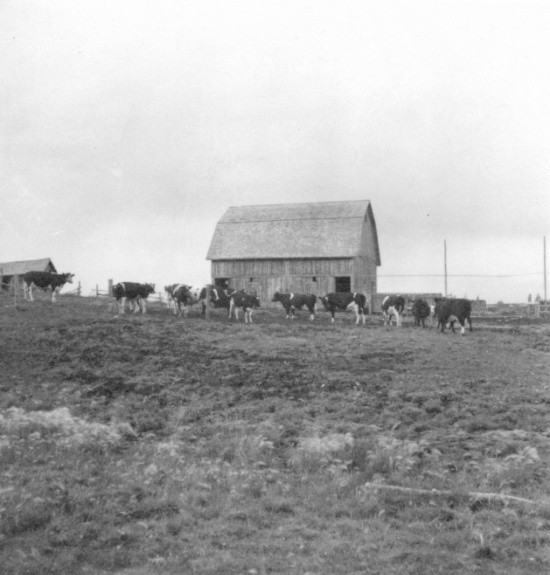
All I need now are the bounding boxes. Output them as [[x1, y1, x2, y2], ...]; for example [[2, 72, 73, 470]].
[[195, 287, 244, 319], [113, 282, 155, 316], [271, 291, 318, 321], [319, 292, 365, 325], [429, 299, 473, 336], [164, 284, 180, 314], [431, 296, 457, 329], [169, 283, 199, 317], [228, 293, 261, 323], [382, 295, 405, 325], [22, 271, 74, 304], [410, 298, 431, 328]]
